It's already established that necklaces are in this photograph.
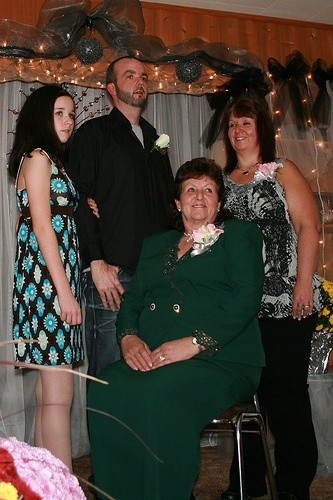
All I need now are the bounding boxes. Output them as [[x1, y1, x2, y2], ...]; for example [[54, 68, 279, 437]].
[[237, 161, 260, 176], [185, 233, 194, 242]]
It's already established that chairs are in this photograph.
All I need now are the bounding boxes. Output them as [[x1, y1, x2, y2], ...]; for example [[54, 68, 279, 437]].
[[203, 392, 278, 500]]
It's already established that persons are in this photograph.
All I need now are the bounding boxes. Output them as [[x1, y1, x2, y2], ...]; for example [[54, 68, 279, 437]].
[[87, 157, 266, 500], [221, 98, 319, 500], [66, 56, 185, 386], [6, 84, 101, 473]]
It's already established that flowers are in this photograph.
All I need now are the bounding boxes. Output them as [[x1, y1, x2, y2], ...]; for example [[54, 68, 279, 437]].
[[315, 277, 333, 332], [187, 222, 225, 244], [258, 162, 284, 176], [0, 435, 88, 499], [150, 134, 171, 156]]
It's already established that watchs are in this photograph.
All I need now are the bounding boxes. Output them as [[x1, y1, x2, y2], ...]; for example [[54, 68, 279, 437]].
[[191, 335, 203, 352]]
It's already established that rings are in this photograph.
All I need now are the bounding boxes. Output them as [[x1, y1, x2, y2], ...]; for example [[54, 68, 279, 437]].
[[304, 305, 311, 309], [160, 356, 166, 360]]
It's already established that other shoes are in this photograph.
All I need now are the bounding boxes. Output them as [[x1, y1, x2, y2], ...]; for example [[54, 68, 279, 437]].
[[220, 489, 252, 500], [271, 492, 310, 500]]
